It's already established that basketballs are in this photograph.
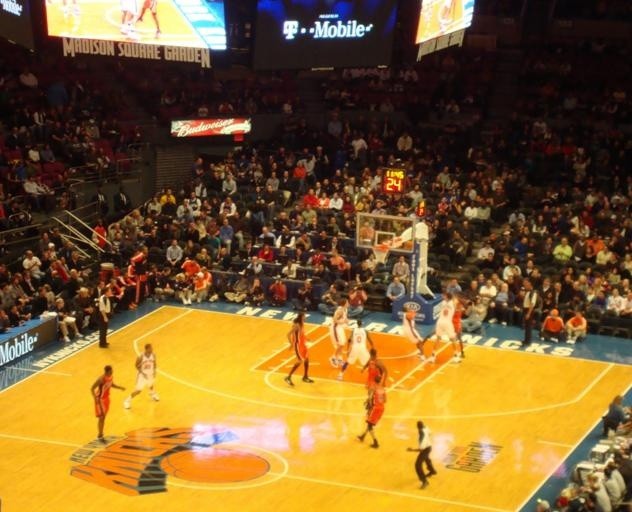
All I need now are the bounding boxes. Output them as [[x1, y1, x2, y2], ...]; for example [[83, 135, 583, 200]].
[[404, 241, 413, 249]]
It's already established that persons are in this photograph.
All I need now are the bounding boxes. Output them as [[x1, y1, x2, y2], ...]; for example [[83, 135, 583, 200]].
[[118, 1, 139, 41], [135, 0, 165, 40], [284, 313, 315, 385], [421, 0, 457, 37], [533, 392, 632, 510], [406, 422, 437, 487], [90, 364, 126, 445], [123, 343, 161, 409], [63, 0, 81, 15], [357, 375, 386, 447], [0, 38, 630, 380], [358, 348, 388, 409]]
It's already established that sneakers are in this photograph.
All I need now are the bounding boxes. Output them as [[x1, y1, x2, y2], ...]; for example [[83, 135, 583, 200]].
[[286, 356, 345, 386], [104, 342, 110, 345], [75, 332, 83, 337], [420, 481, 429, 488], [371, 444, 378, 447], [153, 395, 159, 401], [150, 284, 310, 311], [119, 23, 161, 40], [63, 336, 70, 342], [123, 401, 129, 410], [358, 435, 364, 441], [426, 470, 436, 476], [63, 6, 69, 16], [486, 317, 578, 347], [99, 434, 105, 442], [71, 5, 80, 16], [417, 351, 465, 364], [100, 344, 107, 348]]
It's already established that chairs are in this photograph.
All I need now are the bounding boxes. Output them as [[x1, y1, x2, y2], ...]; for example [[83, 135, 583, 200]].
[[0, 53, 632, 338]]
